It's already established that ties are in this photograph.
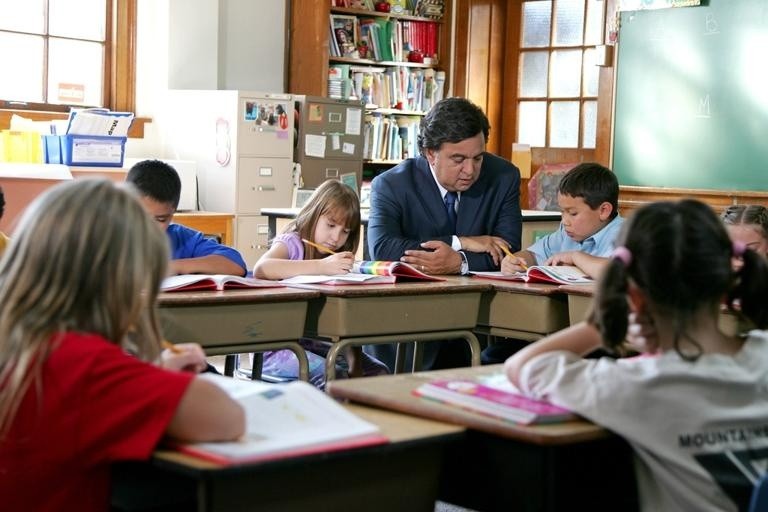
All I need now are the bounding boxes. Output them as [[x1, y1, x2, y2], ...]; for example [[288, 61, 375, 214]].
[[444, 192, 459, 223]]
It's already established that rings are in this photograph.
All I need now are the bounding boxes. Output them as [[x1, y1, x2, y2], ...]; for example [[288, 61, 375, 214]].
[[421, 265, 425, 273]]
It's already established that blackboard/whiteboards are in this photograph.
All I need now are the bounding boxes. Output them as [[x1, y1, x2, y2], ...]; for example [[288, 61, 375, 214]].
[[608, 0, 768, 198]]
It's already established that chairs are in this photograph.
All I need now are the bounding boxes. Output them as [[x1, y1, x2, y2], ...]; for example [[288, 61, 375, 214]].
[[235, 350, 295, 383]]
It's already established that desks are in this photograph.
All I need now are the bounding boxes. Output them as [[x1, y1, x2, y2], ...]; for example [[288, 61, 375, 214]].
[[469, 270, 570, 340], [323, 361, 633, 511], [259, 204, 564, 250], [558, 281, 597, 327], [170, 211, 235, 246], [294, 276, 495, 381], [151, 277, 319, 385], [152, 368, 470, 511]]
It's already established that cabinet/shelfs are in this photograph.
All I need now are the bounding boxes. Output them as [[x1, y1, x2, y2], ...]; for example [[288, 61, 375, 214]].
[[155, 90, 297, 272], [269, 94, 365, 208], [288, 0, 456, 176]]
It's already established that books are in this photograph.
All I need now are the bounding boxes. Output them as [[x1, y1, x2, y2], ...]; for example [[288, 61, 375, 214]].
[[328, 0, 447, 162], [156, 270, 287, 291], [164, 371, 390, 467], [468, 264, 598, 285], [286, 259, 448, 287], [412, 374, 589, 426]]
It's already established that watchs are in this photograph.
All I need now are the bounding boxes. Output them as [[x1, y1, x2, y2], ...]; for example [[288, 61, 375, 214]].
[[459, 252, 469, 276]]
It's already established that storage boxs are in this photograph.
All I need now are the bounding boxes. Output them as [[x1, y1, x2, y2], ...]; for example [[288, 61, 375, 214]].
[[1, 131, 46, 165], [41, 132, 128, 168]]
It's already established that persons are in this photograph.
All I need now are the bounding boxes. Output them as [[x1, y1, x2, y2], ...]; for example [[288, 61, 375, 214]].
[[505, 200, 768, 511], [1, 176, 247, 512], [479, 162, 627, 366], [247, 179, 390, 401], [717, 205, 768, 272], [361, 96, 522, 374], [0, 185, 13, 262], [122, 160, 247, 376]]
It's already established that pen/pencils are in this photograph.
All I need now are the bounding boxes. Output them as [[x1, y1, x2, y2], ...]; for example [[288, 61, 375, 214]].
[[499, 244, 528, 271], [301, 238, 337, 255], [162, 340, 208, 368]]
